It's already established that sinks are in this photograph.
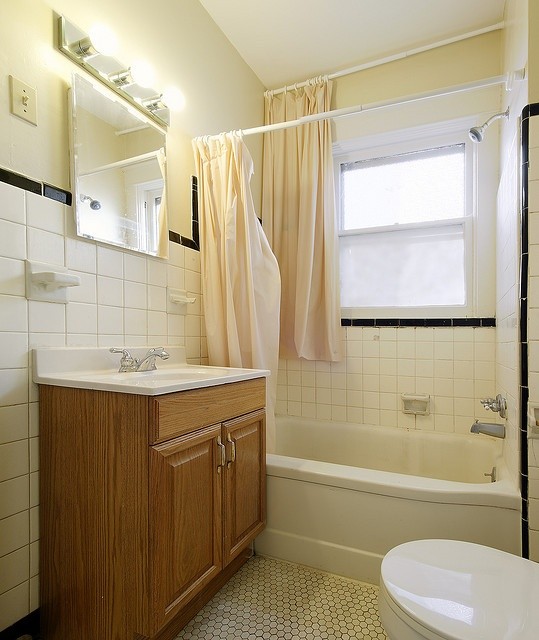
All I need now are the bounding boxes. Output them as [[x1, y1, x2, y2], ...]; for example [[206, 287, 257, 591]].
[[117, 371, 229, 383]]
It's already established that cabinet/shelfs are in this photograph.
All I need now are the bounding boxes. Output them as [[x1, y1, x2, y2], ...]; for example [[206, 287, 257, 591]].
[[34, 389, 266, 637]]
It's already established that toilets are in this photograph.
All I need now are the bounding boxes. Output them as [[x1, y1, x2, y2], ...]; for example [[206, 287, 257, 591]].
[[378, 538, 538, 640]]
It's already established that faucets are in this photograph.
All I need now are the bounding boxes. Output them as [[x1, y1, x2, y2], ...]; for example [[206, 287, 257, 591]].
[[470, 421, 506, 440], [135, 349, 169, 371]]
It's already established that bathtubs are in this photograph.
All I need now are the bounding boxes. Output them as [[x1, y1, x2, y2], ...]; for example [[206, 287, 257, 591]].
[[265, 417, 520, 580]]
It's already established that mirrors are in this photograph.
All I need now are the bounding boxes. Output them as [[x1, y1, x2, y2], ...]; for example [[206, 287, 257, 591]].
[[71, 71, 169, 260]]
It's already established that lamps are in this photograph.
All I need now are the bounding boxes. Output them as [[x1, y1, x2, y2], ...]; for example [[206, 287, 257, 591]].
[[79, 22, 118, 58], [119, 57, 156, 88], [151, 85, 184, 110]]
